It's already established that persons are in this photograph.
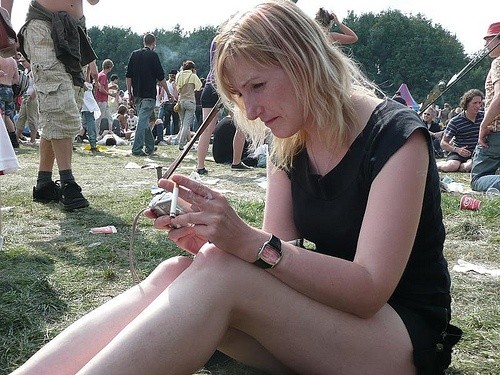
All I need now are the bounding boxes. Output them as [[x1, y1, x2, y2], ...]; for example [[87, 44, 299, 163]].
[[0, 0, 264, 210], [313, 7, 360, 46], [393, 89, 487, 172], [7, 0, 461, 375], [471, 19, 500, 193]]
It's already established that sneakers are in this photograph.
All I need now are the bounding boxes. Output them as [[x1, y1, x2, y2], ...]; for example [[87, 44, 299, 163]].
[[18, 136, 30, 143], [27, 140, 40, 146], [158, 141, 168, 146], [230, 161, 253, 171], [197, 167, 209, 176], [10, 141, 19, 148], [32, 179, 91, 210]]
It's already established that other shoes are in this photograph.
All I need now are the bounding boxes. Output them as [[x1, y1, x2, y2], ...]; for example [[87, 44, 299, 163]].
[[90, 148, 100, 154], [179, 146, 184, 151], [154, 146, 158, 152], [141, 153, 149, 156]]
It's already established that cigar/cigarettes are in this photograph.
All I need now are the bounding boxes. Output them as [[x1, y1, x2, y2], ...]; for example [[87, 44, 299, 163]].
[[169, 181, 180, 220]]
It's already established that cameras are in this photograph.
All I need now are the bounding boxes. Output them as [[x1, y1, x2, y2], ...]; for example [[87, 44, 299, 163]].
[[148, 191, 195, 230]]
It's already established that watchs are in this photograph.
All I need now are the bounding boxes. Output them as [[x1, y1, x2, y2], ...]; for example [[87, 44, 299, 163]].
[[252, 232, 285, 270]]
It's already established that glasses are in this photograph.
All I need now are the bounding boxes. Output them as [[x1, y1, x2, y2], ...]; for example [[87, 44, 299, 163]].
[[423, 112, 431, 116]]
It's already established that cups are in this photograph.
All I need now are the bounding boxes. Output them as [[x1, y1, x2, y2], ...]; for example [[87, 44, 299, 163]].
[[486, 188, 499, 201], [460, 195, 482, 211]]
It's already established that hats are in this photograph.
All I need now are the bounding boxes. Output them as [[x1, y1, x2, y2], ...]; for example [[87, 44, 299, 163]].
[[483, 21, 500, 38]]
